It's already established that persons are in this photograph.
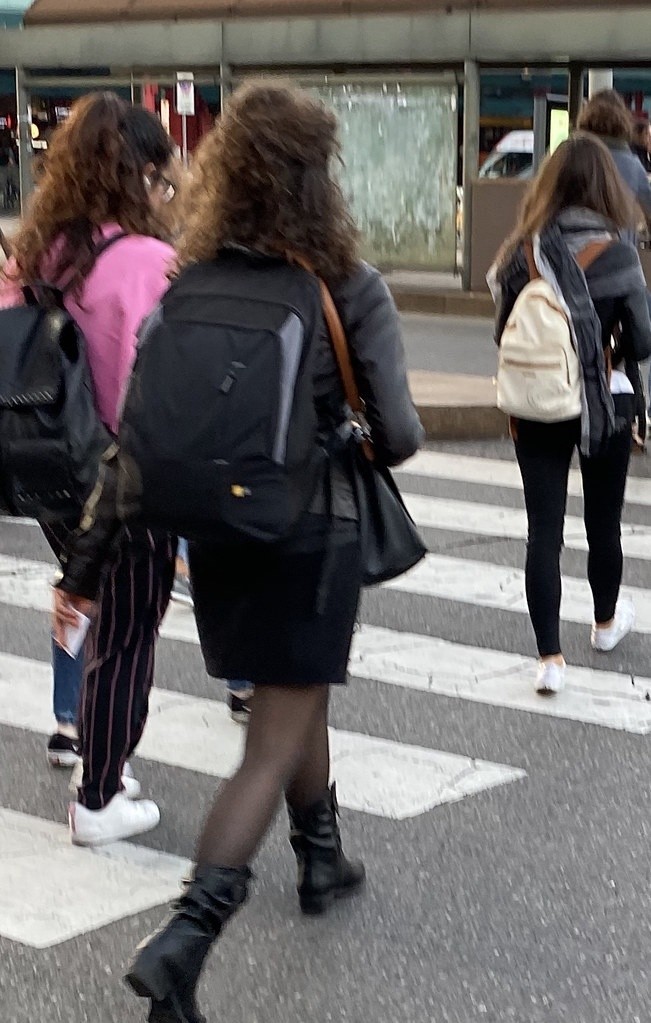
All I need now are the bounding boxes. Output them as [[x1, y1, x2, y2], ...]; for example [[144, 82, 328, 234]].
[[575, 86, 651, 449], [484, 130, 651, 696], [55, 76, 426, 1022], [0, 92, 184, 848]]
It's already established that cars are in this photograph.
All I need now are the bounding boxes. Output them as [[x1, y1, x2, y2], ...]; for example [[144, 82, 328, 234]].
[[478, 129, 535, 179]]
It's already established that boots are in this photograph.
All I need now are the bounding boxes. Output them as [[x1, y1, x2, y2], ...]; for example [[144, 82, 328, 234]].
[[283, 779, 366, 915], [123, 858, 259, 1022]]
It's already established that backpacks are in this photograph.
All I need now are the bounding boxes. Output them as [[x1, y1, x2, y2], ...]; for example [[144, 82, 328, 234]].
[[116, 239, 347, 555], [493, 228, 619, 421], [0, 229, 150, 519]]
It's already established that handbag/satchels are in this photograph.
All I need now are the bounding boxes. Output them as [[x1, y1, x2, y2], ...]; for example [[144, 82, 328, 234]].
[[289, 248, 427, 587]]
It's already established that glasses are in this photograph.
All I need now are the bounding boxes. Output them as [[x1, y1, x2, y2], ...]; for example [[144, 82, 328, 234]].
[[155, 170, 177, 204]]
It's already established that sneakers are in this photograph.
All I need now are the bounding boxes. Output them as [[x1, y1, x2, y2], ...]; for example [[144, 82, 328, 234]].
[[46, 732, 81, 767], [534, 657, 566, 694], [66, 757, 141, 798], [67, 789, 160, 846], [590, 598, 634, 651], [223, 687, 255, 724]]
